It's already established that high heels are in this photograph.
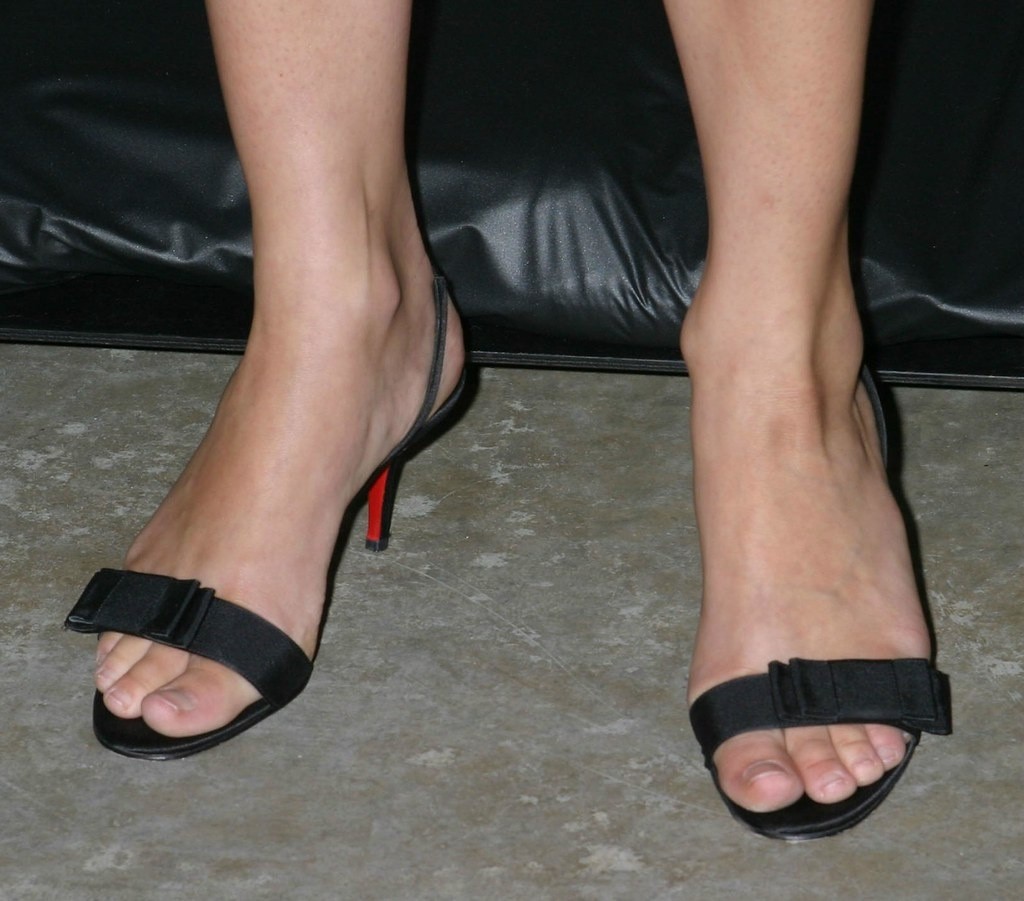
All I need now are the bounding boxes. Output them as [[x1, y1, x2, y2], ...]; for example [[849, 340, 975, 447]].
[[688, 348, 955, 842], [63, 274, 479, 762]]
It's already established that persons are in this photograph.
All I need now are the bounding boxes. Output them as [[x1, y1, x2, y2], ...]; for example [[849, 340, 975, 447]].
[[60, 0, 951, 841]]
